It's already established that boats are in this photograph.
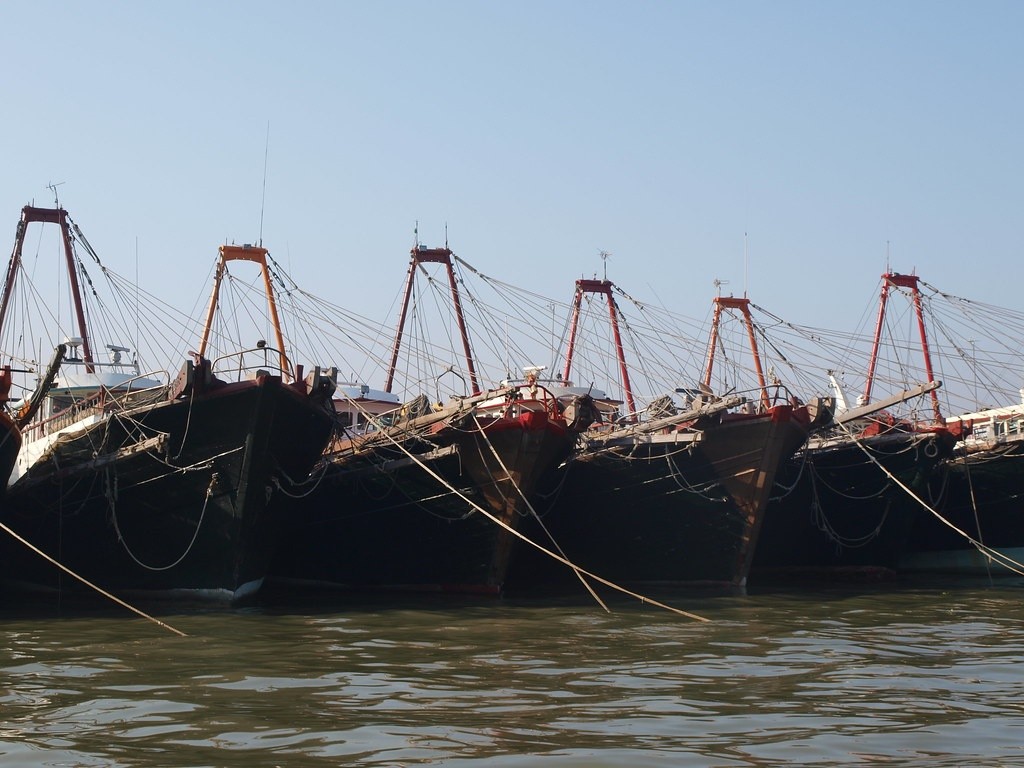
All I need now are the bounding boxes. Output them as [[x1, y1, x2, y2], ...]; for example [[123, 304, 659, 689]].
[[0, 181, 344, 614], [353, 223, 837, 601], [542, 251, 975, 601], [160, 244, 598, 602], [672, 284, 1024, 598]]
